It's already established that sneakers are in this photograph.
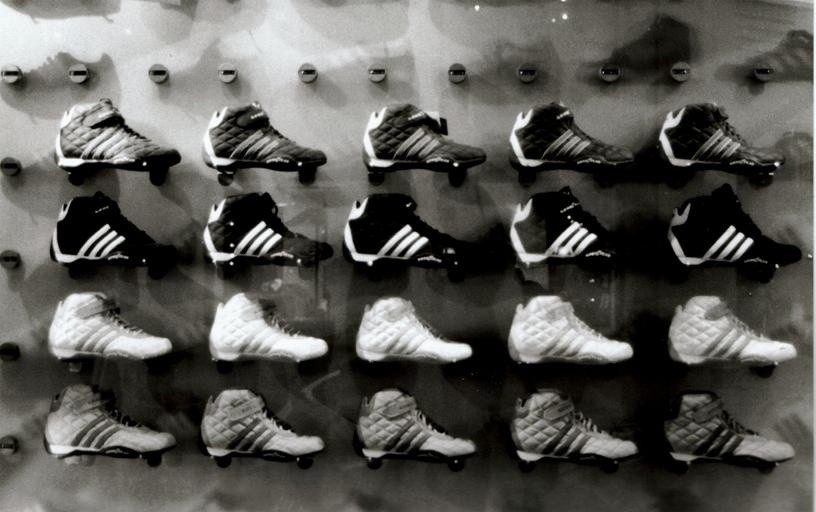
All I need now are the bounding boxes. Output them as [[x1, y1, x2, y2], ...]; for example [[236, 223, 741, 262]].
[[508, 102, 635, 170], [356, 297, 472, 365], [509, 388, 637, 459], [48, 292, 172, 362], [49, 189, 189, 267], [200, 389, 323, 460], [202, 191, 335, 266], [53, 99, 181, 172], [665, 391, 795, 460], [354, 389, 477, 458], [664, 183, 802, 269], [199, 102, 328, 170], [611, 15, 691, 64], [667, 296, 798, 366], [207, 293, 328, 363], [750, 32, 814, 78], [362, 104, 487, 174], [44, 383, 177, 458], [655, 104, 786, 175], [507, 187, 635, 264], [506, 295, 633, 364], [342, 193, 477, 268]]
